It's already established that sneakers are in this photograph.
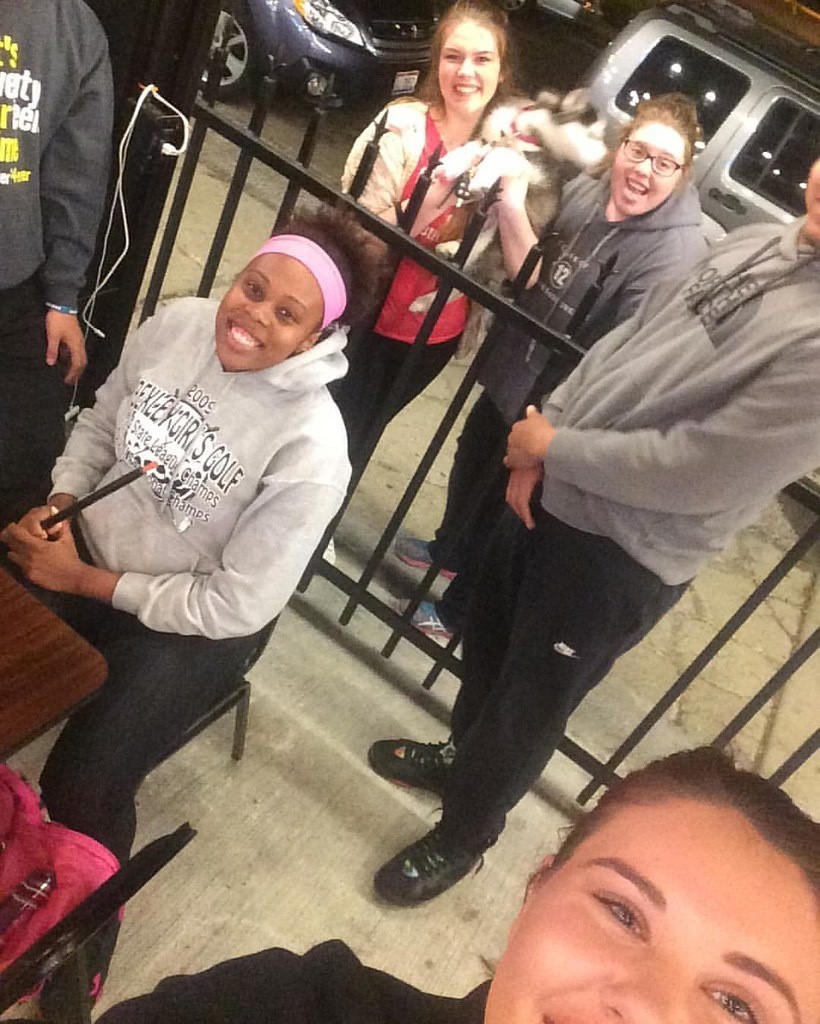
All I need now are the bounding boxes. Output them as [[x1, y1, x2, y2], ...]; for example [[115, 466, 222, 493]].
[[369, 733, 460, 798], [321, 536, 336, 565], [373, 809, 498, 907], [401, 596, 455, 639], [395, 536, 457, 577]]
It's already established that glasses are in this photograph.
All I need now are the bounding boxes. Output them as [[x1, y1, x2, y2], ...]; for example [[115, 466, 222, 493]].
[[624, 137, 685, 177]]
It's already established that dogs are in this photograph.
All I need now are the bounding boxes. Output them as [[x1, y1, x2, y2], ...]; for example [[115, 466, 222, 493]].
[[418, 86, 610, 208]]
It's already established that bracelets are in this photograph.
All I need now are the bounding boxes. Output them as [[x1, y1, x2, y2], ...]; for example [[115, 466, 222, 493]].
[[46, 302, 78, 314]]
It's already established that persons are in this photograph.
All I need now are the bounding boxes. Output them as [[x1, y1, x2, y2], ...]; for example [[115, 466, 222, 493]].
[[0, 0, 114, 527], [367, 159, 820, 907], [96, 746, 820, 1024], [0, 207, 375, 1024], [323, 5, 707, 639]]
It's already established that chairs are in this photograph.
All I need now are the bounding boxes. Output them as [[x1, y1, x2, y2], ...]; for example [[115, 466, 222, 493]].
[[134, 323, 386, 804]]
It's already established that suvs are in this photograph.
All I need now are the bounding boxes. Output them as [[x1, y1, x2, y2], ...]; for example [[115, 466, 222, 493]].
[[573, 2, 820, 232]]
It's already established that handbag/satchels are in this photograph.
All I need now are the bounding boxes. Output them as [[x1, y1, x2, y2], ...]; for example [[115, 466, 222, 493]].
[[0, 763, 124, 1004]]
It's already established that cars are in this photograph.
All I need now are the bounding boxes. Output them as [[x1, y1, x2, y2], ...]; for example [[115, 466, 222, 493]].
[[197, 0, 444, 119], [501, 1, 630, 38]]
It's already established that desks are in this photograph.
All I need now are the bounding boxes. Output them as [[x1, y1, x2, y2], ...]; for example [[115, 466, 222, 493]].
[[0, 570, 110, 764]]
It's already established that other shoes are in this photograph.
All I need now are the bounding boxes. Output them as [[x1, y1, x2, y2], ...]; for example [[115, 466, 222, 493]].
[[40, 906, 123, 1020]]
